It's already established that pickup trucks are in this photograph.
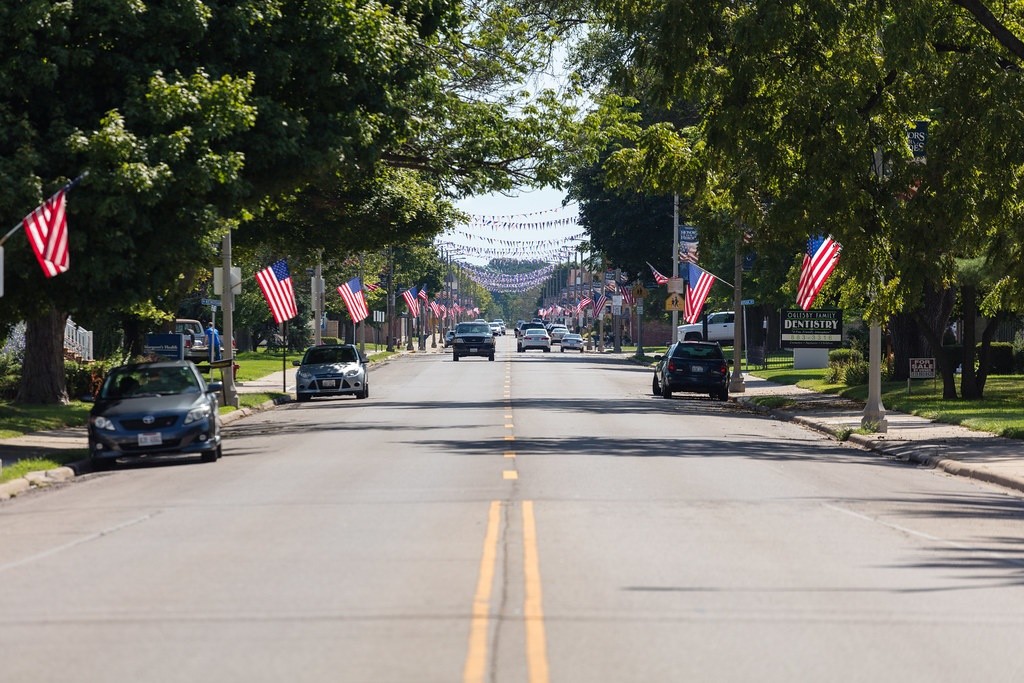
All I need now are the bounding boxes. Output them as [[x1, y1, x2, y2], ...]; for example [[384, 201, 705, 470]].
[[677, 310, 768, 348]]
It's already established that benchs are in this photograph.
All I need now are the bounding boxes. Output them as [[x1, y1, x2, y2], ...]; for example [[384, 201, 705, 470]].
[[676, 350, 719, 359]]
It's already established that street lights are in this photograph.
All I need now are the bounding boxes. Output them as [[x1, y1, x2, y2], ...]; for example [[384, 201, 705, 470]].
[[446, 248, 461, 332], [559, 250, 578, 334], [439, 241, 455, 345], [569, 235, 592, 350], [562, 245, 583, 303], [450, 252, 465, 328], [553, 254, 570, 329]]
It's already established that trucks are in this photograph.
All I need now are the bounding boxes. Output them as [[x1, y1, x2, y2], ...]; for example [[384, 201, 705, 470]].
[[167, 316, 238, 366]]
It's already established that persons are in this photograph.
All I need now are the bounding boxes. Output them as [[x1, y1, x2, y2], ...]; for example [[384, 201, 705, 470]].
[[203, 322, 222, 362]]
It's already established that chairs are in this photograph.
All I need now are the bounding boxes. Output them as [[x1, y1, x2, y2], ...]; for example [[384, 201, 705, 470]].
[[118, 377, 140, 396], [342, 350, 355, 362]]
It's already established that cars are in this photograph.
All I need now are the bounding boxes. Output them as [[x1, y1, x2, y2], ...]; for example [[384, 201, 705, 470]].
[[443, 330, 456, 348], [292, 344, 370, 401], [560, 333, 585, 353], [454, 317, 570, 353], [520, 328, 553, 353], [79, 358, 223, 465]]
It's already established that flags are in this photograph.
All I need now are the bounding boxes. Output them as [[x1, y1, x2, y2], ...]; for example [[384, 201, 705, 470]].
[[795, 226, 843, 310], [683, 263, 715, 324], [615, 281, 636, 304], [23, 189, 69, 278], [538, 292, 607, 320], [401, 284, 480, 321], [255, 257, 298, 324], [650, 266, 669, 285], [335, 276, 370, 324]]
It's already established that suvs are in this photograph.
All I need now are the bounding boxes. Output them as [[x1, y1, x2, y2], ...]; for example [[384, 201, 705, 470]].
[[449, 321, 496, 362], [652, 338, 735, 402]]
